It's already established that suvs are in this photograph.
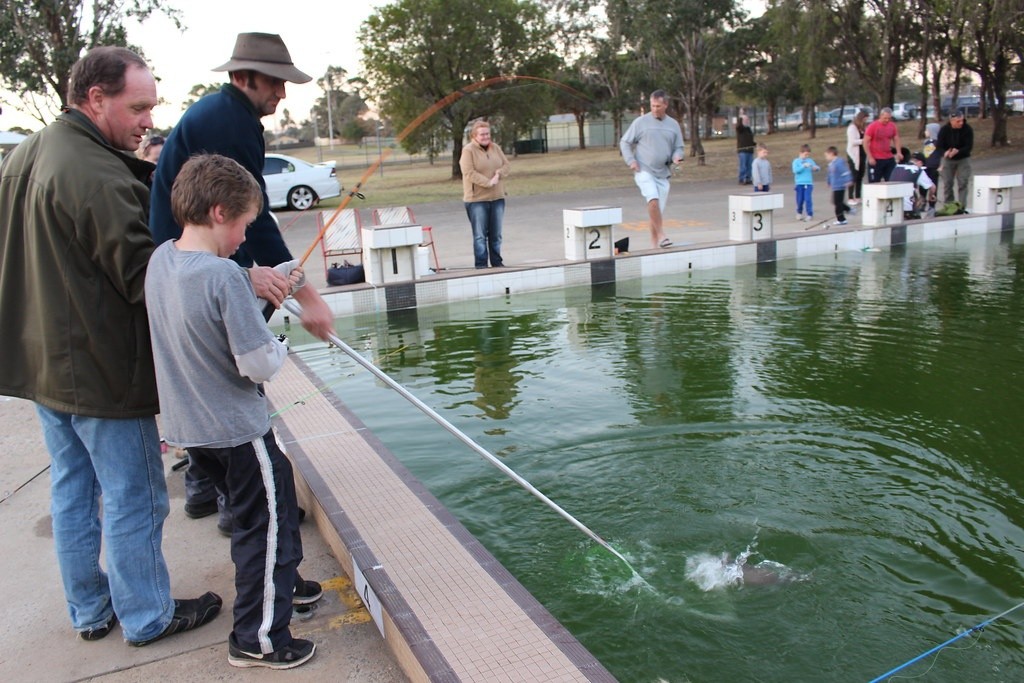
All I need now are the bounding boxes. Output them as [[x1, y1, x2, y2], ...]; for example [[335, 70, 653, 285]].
[[943, 94, 1019, 117]]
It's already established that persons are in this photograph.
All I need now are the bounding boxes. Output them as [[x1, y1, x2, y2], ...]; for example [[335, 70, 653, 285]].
[[863, 108, 903, 183], [792, 144, 820, 221], [137, 136, 166, 164], [148, 32, 332, 536], [458, 121, 511, 269], [918, 123, 941, 211], [143, 155, 322, 669], [890, 152, 936, 219], [619, 90, 685, 246], [0, 45, 291, 646], [847, 112, 870, 206], [937, 110, 974, 208], [736, 108, 758, 185], [825, 146, 856, 225], [752, 145, 772, 192]]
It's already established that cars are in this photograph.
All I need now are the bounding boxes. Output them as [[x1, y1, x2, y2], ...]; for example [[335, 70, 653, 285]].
[[775, 101, 942, 132], [260, 153, 342, 215]]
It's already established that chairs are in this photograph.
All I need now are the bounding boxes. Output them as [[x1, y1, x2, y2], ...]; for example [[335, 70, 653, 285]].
[[317, 208, 363, 281], [372, 206, 440, 272]]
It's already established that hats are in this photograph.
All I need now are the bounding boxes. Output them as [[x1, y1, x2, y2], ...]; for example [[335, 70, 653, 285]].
[[211, 32, 314, 84]]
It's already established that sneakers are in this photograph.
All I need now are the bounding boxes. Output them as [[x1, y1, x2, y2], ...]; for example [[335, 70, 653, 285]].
[[291, 580, 323, 605], [228, 631, 316, 670]]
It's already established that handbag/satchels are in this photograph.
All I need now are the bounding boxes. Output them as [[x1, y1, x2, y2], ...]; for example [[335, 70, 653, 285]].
[[326, 261, 365, 287], [934, 201, 968, 215]]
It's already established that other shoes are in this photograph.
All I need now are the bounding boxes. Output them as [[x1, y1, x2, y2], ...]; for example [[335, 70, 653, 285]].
[[746, 181, 753, 185], [796, 212, 803, 220], [737, 182, 744, 186], [182, 504, 217, 519], [848, 198, 861, 205], [804, 215, 813, 223], [122, 590, 224, 646], [80, 613, 116, 641], [215, 507, 306, 538], [834, 220, 848, 225]]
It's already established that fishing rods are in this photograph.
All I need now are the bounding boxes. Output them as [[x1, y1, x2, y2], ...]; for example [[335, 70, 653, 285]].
[[253, 75, 599, 324]]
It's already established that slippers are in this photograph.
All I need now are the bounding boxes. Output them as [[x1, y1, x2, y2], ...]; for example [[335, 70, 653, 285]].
[[658, 238, 673, 248]]
